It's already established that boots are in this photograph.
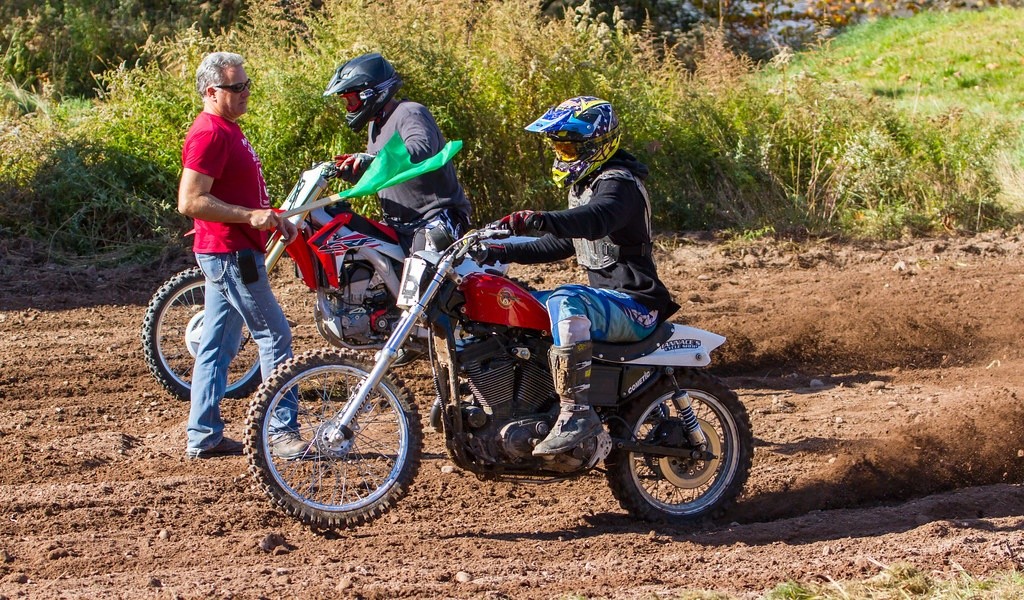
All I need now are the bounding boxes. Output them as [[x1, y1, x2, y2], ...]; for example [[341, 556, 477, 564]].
[[531, 340, 603, 456]]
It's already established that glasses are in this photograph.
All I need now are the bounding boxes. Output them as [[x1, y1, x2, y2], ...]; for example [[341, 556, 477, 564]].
[[340, 93, 362, 112], [549, 138, 578, 161], [214, 78, 251, 93]]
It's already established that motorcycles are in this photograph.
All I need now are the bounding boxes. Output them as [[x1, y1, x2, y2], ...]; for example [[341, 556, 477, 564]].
[[242, 221, 760, 535], [140, 154, 521, 404]]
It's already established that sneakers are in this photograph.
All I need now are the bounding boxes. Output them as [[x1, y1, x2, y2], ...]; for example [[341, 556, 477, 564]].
[[189, 437, 247, 456], [272, 436, 308, 459]]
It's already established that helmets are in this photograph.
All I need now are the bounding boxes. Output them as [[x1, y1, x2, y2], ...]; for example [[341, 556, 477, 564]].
[[322, 53, 399, 133], [524, 97, 621, 190]]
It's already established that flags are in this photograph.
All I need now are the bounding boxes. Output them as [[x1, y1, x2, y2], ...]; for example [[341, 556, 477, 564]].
[[339, 130, 463, 200]]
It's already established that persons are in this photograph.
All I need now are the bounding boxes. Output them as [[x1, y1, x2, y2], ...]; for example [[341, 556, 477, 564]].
[[179, 52, 319, 458], [471, 95, 681, 454], [324, 52, 470, 267]]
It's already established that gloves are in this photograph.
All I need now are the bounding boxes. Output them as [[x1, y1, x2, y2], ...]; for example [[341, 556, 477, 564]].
[[331, 171, 363, 185], [495, 210, 542, 236], [333, 152, 375, 175], [488, 243, 506, 267]]
